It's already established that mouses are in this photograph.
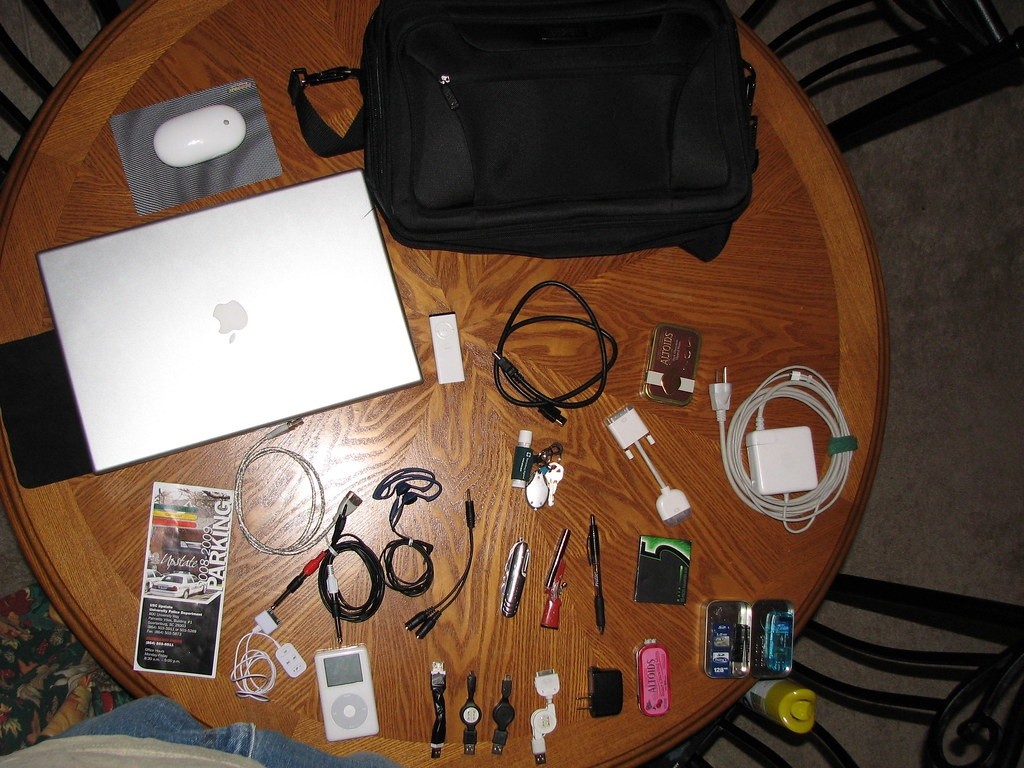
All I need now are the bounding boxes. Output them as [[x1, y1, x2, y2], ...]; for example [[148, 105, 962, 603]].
[[153, 105, 247, 169]]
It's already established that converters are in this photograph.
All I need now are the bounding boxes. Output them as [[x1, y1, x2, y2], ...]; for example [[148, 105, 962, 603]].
[[745, 426, 818, 496]]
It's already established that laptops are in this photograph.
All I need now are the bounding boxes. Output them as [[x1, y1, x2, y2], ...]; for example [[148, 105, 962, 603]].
[[36, 167, 423, 475]]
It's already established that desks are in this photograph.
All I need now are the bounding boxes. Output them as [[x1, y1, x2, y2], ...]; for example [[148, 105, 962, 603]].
[[0, 36, 1024, 768]]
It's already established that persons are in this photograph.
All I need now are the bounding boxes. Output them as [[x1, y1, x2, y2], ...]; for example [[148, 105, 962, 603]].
[[0, 695, 410, 768]]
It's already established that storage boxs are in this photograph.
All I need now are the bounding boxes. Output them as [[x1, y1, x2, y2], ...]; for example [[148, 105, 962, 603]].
[[634, 535, 693, 607], [635, 644, 671, 717], [640, 323, 701, 407]]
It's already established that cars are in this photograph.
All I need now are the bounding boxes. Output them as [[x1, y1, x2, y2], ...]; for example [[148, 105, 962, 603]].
[[149, 571, 209, 599], [145, 568, 167, 593]]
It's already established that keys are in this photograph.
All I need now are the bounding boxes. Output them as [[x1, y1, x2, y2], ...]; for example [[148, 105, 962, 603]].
[[545, 462, 563, 507]]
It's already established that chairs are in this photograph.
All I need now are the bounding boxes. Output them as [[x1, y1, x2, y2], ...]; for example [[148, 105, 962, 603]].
[[654, 575, 1024, 767], [739, 0, 1024, 150], [0, 0, 121, 188]]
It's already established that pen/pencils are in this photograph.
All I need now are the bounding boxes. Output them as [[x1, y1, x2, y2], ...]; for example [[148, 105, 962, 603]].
[[586, 513, 606, 634]]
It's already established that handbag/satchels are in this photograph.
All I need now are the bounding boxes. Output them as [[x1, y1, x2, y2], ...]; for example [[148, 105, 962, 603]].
[[288, 0, 759, 263]]
